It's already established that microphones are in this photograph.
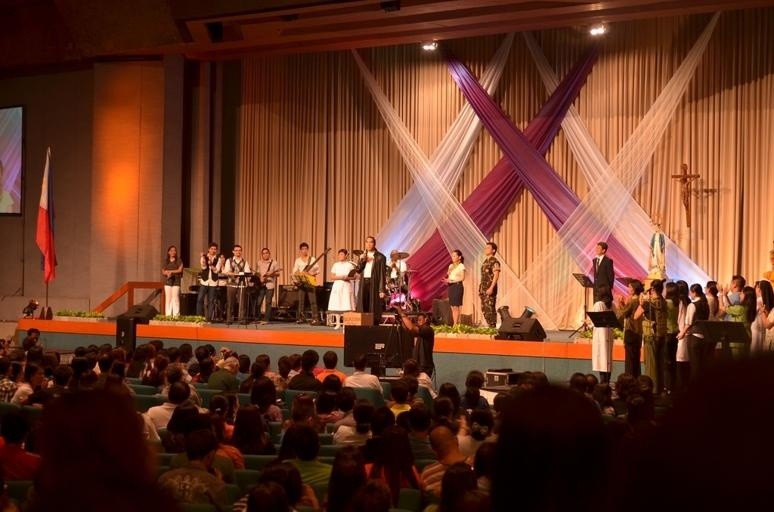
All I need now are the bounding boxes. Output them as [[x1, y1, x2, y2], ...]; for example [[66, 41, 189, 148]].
[[418, 321, 426, 328], [661, 278, 666, 284], [364, 248, 368, 256]]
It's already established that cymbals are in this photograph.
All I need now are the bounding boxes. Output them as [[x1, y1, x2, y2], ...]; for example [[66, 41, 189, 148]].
[[403, 270, 417, 273], [391, 253, 410, 260], [351, 250, 363, 254]]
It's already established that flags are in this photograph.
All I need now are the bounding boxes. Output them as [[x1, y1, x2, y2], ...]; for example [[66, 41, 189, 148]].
[[31, 144, 60, 286]]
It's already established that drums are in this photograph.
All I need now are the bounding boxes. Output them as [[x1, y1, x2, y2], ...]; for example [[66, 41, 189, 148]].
[[385, 265, 392, 278]]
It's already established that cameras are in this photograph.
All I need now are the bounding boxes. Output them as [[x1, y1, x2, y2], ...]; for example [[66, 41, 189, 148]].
[[389, 301, 405, 314]]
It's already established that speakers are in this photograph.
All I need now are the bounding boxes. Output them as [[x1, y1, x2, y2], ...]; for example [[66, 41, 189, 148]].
[[278, 285, 299, 311], [115, 317, 136, 351], [125, 304, 159, 325], [179, 293, 198, 316], [497, 318, 547, 341]]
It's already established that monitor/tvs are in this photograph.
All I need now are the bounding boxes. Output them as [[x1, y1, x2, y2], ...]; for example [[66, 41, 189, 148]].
[[343, 324, 415, 377]]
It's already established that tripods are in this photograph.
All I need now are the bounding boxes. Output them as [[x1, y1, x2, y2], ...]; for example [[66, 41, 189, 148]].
[[377, 314, 404, 380], [568, 287, 593, 338]]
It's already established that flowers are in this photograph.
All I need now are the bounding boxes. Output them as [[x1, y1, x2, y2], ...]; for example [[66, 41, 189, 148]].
[[578, 328, 623, 339], [152, 314, 208, 323], [55, 308, 104, 319], [430, 325, 498, 335]]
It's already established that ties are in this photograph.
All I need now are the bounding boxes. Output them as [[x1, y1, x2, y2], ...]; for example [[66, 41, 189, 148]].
[[596, 258, 600, 273]]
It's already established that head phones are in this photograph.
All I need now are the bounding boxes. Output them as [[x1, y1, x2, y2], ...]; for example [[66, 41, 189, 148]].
[[420, 312, 431, 323]]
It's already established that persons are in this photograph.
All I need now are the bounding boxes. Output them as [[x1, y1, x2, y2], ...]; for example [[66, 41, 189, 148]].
[[326, 236, 408, 329], [0, 329, 492, 511], [493, 275, 773, 511], [161, 246, 183, 317], [292, 242, 320, 324], [478, 243, 500, 331], [392, 304, 435, 378], [592, 242, 615, 308], [197, 242, 278, 325], [673, 174, 701, 212], [442, 249, 466, 328]]
